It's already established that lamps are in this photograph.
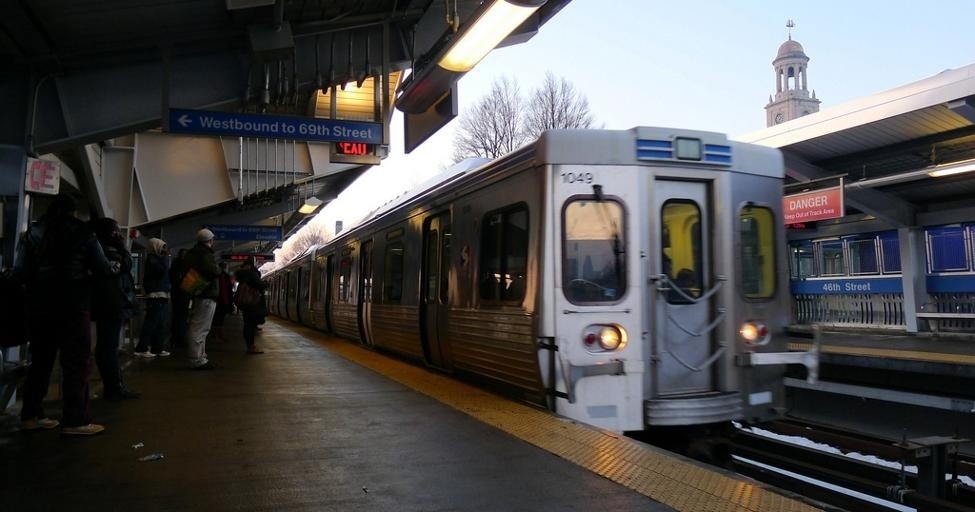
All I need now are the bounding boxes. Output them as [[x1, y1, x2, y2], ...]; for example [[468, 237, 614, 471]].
[[396, 1, 542, 114]]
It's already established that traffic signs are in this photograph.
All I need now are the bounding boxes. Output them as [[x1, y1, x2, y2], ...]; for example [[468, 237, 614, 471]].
[[164, 107, 384, 144], [203, 225, 283, 242]]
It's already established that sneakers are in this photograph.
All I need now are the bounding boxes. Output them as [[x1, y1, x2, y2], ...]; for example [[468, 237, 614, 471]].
[[132, 350, 171, 358], [189, 361, 219, 371], [246, 344, 265, 353], [103, 381, 143, 400], [61, 424, 105, 435], [19, 415, 60, 429]]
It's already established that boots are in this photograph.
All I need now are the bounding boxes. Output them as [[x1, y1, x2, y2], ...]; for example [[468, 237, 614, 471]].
[[209, 325, 232, 344]]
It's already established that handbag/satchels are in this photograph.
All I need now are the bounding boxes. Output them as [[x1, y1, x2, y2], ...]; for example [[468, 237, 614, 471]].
[[233, 279, 261, 312], [178, 269, 211, 299]]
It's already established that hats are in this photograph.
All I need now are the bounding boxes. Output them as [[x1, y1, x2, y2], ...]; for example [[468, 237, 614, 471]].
[[197, 228, 215, 243]]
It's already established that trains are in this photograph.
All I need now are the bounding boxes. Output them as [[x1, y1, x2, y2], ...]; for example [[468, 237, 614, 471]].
[[263, 126, 812, 437]]
